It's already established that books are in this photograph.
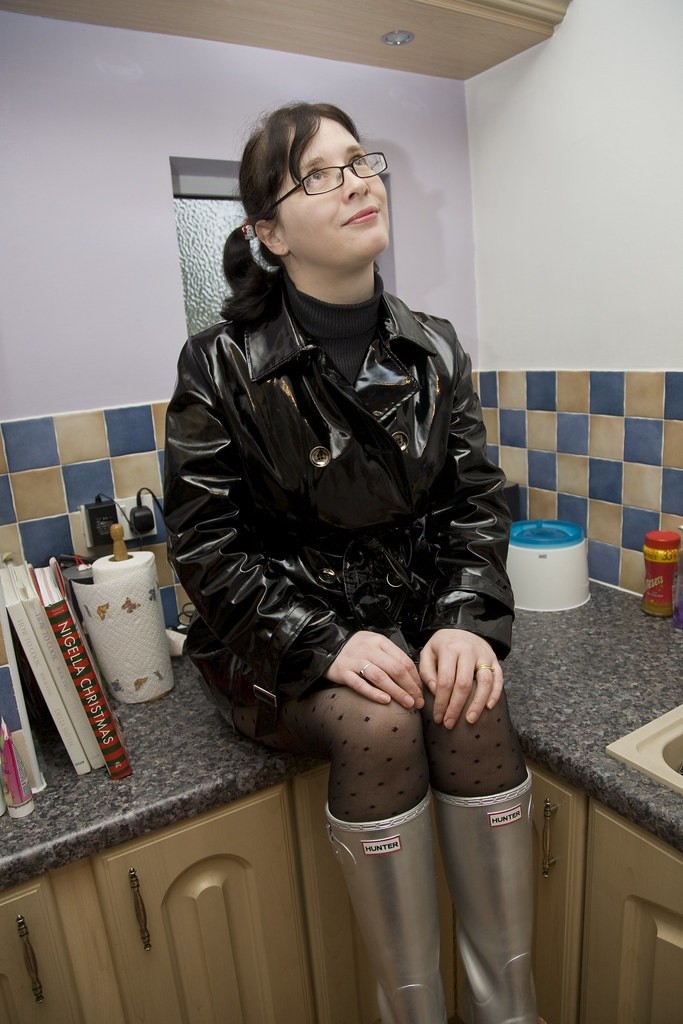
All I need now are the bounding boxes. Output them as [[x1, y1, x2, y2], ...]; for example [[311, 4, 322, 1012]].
[[1, 557, 134, 820]]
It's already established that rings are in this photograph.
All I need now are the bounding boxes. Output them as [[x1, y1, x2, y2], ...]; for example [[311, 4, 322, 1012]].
[[359, 662, 371, 677], [475, 664, 495, 672]]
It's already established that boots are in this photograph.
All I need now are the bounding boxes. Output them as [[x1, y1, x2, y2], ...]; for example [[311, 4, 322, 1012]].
[[433, 767, 544, 1024], [325, 784, 446, 1024]]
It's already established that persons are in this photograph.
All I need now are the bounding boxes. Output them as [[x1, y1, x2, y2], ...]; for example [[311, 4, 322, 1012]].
[[160, 101, 547, 1024]]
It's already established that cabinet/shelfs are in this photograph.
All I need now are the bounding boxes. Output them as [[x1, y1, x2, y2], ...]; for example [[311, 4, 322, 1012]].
[[455, 763, 683, 1024], [290, 768, 435, 1024], [0, 784, 315, 1024]]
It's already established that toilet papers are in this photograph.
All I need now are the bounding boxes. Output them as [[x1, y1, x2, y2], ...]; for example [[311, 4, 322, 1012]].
[[72, 552, 175, 705]]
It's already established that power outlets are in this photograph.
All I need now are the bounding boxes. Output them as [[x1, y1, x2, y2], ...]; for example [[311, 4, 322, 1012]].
[[79, 494, 158, 547]]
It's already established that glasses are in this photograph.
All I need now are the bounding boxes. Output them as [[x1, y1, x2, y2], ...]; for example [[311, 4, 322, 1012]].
[[268, 152, 388, 210]]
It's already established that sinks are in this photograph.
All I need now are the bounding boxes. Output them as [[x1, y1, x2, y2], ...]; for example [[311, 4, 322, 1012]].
[[606, 701, 683, 792]]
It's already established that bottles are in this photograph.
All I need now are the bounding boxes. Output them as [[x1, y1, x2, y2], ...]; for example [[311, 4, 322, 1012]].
[[640, 531, 681, 617]]
